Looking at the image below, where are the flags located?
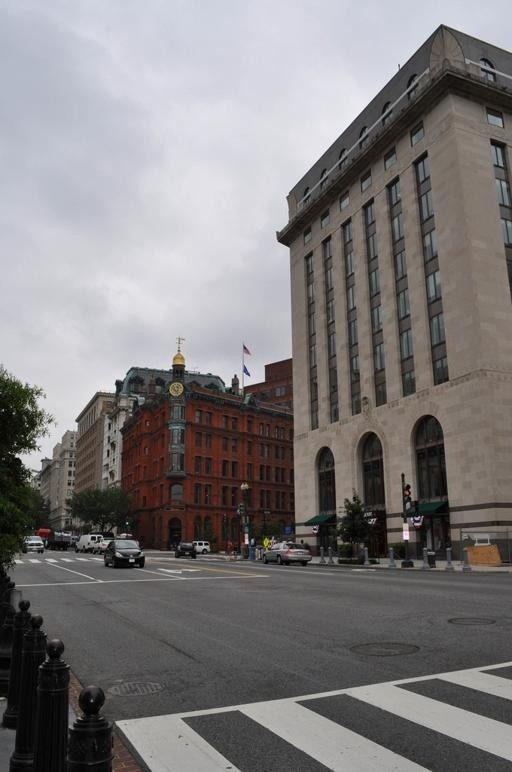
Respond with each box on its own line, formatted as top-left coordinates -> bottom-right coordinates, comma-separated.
243,344 -> 251,377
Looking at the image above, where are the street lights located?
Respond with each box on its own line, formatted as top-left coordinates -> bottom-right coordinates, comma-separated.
239,481 -> 251,558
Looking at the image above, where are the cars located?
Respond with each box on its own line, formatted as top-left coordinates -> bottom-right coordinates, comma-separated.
174,539 -> 210,559
104,540 -> 145,568
263,542 -> 312,566
20,528 -> 113,557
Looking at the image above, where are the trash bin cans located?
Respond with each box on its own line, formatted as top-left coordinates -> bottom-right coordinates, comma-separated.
427,552 -> 436,568
249,547 -> 256,560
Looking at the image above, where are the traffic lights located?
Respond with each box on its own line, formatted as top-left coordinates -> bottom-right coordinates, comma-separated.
403,482 -> 411,503
